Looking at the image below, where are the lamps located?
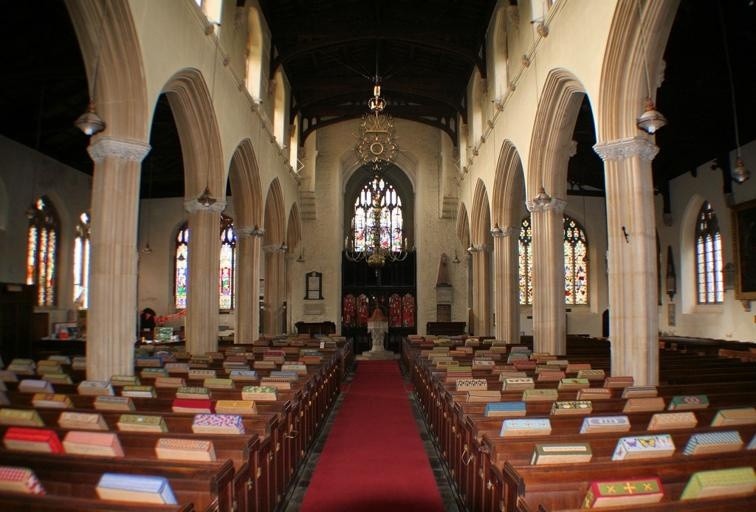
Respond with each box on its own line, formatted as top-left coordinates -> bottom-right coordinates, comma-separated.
525,182 -> 567,213
637,0 -> 666,135
296,224 -> 305,262
488,222 -> 507,239
181,36 -> 227,213
279,241 -> 287,255
466,240 -> 477,254
345,39 -> 416,279
249,101 -> 264,237
74,2 -> 106,136
715,0 -> 750,183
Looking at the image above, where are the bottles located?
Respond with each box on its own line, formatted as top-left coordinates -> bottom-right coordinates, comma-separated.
319,340 -> 325,350
